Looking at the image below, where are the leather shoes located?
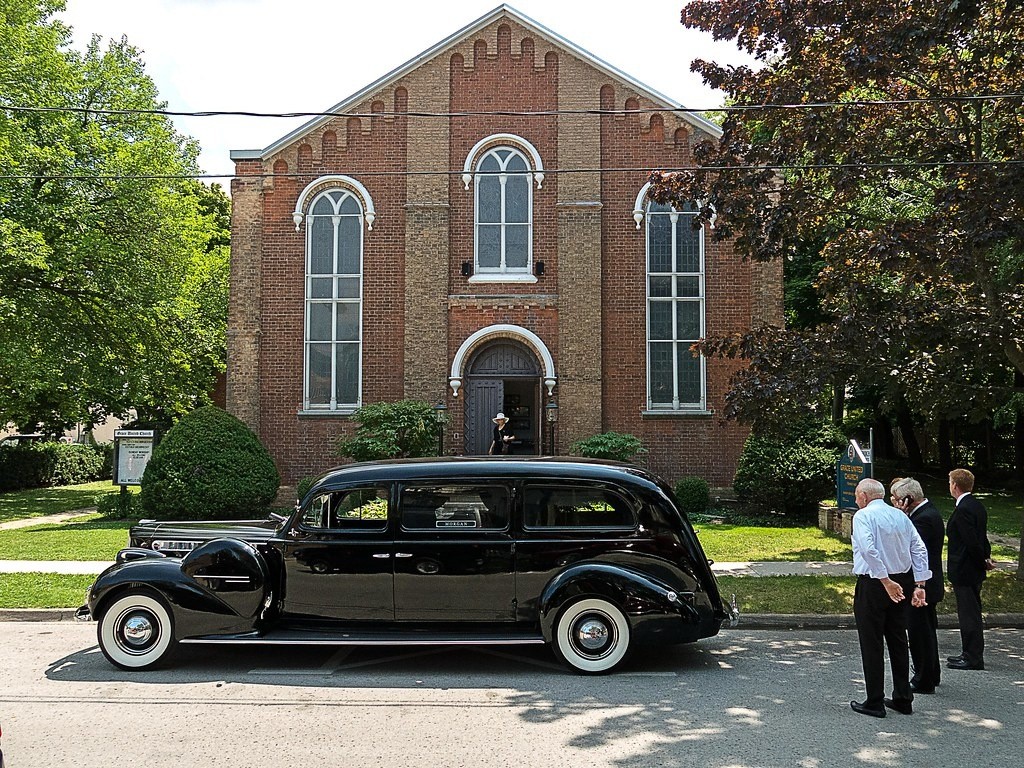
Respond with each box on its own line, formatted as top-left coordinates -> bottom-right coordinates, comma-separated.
948,661 -> 974,670
851,700 -> 886,718
910,664 -> 918,671
909,681 -> 936,694
884,698 -> 903,713
947,655 -> 967,661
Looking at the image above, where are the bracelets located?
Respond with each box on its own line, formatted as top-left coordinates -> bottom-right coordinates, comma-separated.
915,584 -> 925,590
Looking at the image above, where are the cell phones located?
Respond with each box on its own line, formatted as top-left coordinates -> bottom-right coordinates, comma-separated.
902,495 -> 914,508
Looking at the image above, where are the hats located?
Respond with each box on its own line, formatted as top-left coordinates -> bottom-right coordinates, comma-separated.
492,413 -> 509,424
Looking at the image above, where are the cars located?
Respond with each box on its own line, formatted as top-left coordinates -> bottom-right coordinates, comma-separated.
73,453 -> 740,677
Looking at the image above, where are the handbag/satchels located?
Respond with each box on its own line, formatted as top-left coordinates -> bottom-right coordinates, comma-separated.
501,444 -> 509,455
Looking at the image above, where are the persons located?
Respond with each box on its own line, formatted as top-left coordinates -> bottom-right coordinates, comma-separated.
489,412 -> 516,455
946,468 -> 998,670
889,477 -> 946,694
849,478 -> 932,718
